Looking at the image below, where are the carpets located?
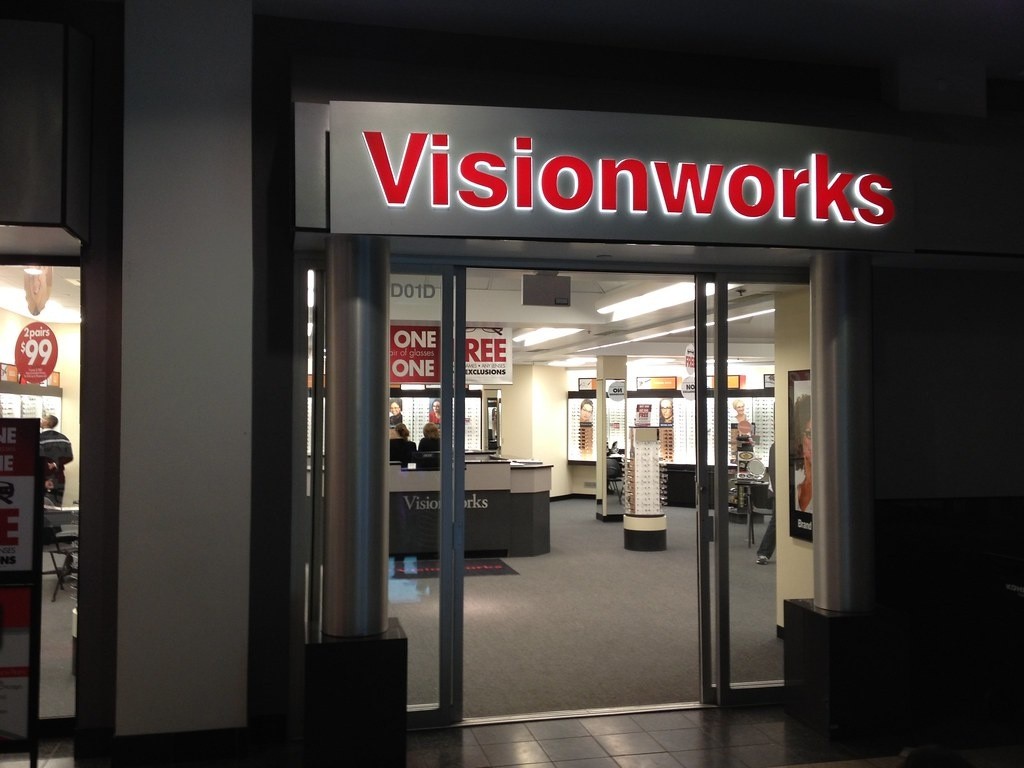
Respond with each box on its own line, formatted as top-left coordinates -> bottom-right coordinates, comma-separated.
387,558 -> 520,580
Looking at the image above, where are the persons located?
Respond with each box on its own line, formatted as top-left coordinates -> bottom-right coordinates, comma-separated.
753,442 -> 775,565
793,392 -> 814,515
731,399 -> 751,424
389,398 -> 404,426
417,422 -> 441,453
429,399 -> 441,439
581,400 -> 593,423
637,404 -> 650,414
389,423 -> 417,453
37,413 -> 74,542
660,398 -> 673,424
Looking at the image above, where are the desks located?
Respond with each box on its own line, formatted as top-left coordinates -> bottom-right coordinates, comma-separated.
44,506 -> 79,526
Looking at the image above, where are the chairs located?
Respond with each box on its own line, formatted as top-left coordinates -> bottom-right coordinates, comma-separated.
43,515 -> 78,602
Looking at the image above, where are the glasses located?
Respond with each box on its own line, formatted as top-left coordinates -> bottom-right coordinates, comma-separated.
677,399 -> 775,462
803,430 -> 811,439
620,407 -> 673,515
569,403 -> 620,455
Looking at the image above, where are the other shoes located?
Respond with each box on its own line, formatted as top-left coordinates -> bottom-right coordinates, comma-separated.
756,556 -> 768,565
